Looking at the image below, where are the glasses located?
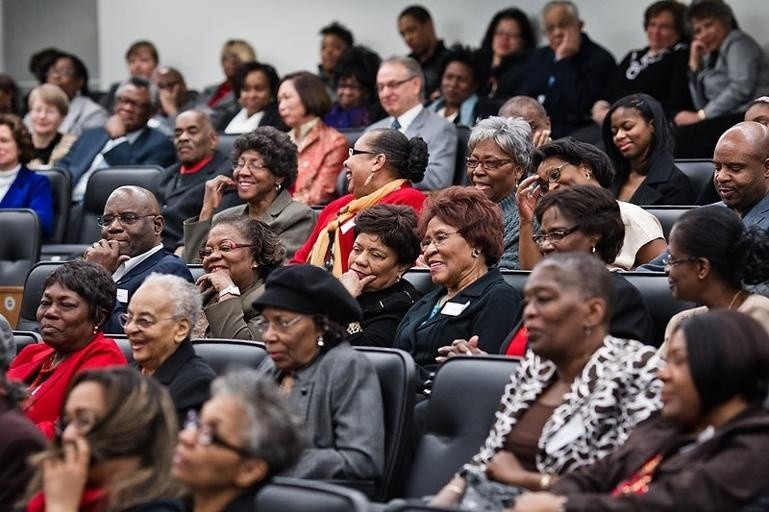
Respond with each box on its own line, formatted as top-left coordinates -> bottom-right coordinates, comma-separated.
374,77 -> 416,90
185,414 -> 242,455
234,162 -> 267,171
250,315 -> 300,332
661,252 -> 697,264
420,230 -> 459,251
200,242 -> 252,257
52,410 -> 97,431
495,30 -> 519,37
96,213 -> 156,228
119,312 -> 169,329
533,224 -> 579,244
535,163 -> 569,196
347,147 -> 374,157
466,158 -> 511,170
542,23 -> 567,29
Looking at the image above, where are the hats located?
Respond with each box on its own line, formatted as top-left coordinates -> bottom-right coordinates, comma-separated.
253,264 -> 363,339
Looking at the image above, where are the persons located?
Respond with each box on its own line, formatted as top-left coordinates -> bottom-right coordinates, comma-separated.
1,2 -> 767,510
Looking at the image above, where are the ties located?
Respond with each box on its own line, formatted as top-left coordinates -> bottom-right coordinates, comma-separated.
391,118 -> 400,130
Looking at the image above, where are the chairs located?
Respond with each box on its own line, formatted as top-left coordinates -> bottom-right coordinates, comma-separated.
353,345 -> 418,502
190,337 -> 267,379
102,334 -> 136,363
406,354 -> 523,500
254,475 -> 364,511
